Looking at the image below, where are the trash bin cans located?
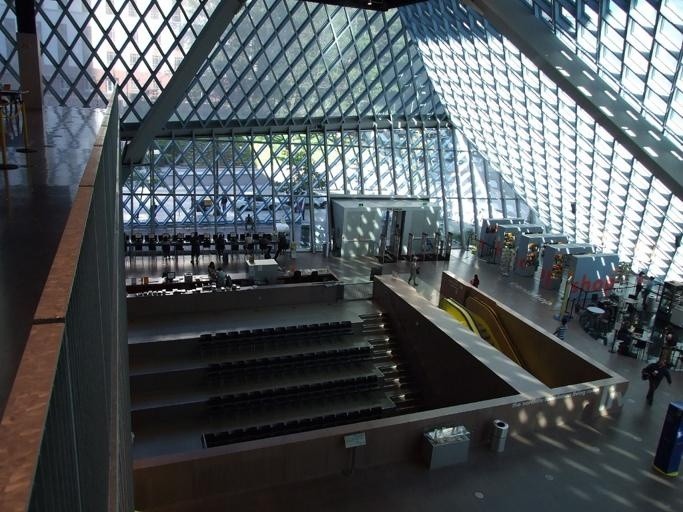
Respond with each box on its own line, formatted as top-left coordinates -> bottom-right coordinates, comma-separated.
323,242 -> 329,257
490,419 -> 509,452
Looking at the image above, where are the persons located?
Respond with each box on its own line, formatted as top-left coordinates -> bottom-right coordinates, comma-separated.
408,256 -> 419,286
617,312 -> 641,347
642,359 -> 672,405
553,318 -> 568,340
472,274 -> 479,287
634,271 -> 654,305
245,214 -> 255,230
243,233 -> 288,260
215,234 -> 225,261
162,237 -> 171,258
208,262 -> 227,288
190,231 -> 200,264
221,197 -> 227,211
659,327 -> 676,365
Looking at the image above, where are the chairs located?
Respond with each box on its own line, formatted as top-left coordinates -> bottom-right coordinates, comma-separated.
199,321 -> 385,450
591,292 -> 637,325
609,327 -> 647,359
293,270 -> 318,282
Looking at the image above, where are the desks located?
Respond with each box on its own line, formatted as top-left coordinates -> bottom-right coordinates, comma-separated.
670,342 -> 683,368
631,335 -> 654,358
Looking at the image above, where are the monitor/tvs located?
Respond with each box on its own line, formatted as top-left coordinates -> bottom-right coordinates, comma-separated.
124,232 -> 272,241
167,272 -> 175,283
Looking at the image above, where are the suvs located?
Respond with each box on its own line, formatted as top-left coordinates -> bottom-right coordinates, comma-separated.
284,190 -> 327,209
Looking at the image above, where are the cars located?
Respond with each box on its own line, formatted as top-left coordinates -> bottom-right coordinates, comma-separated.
235,191 -> 281,211
